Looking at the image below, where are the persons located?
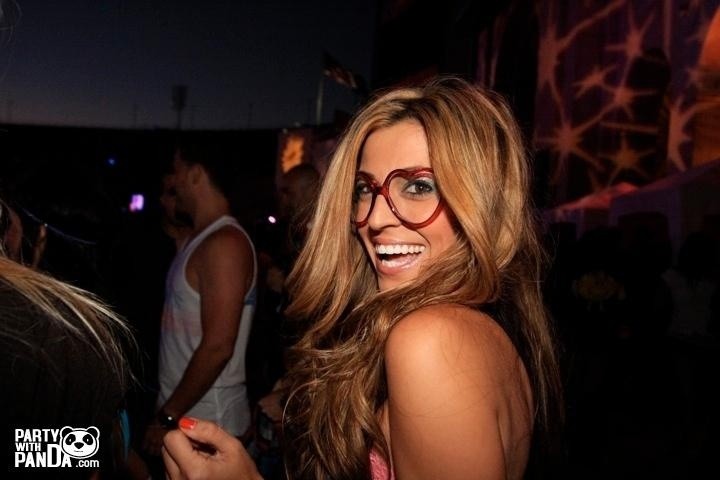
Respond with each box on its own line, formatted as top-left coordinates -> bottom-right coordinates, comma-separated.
159,69 -> 573,480
136,131 -> 340,480
1,240 -> 143,478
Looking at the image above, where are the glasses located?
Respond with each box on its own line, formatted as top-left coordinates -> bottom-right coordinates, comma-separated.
349,168 -> 446,233
